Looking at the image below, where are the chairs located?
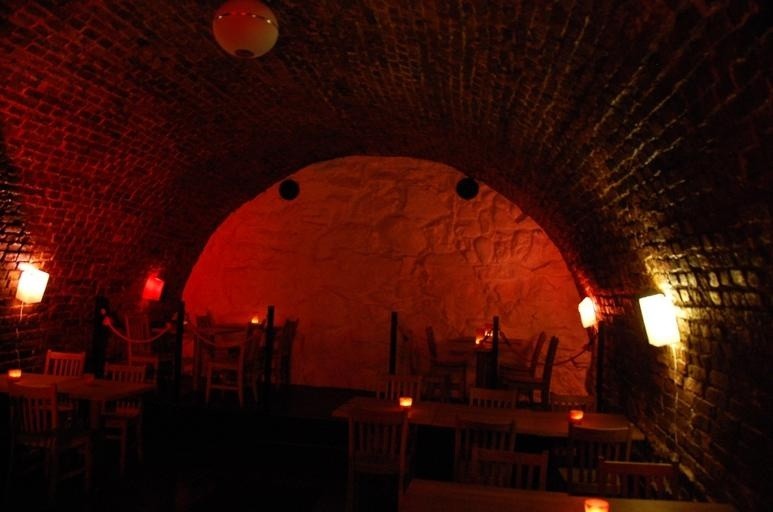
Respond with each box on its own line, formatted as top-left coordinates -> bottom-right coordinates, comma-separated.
446,412 -> 519,486
387,322 -> 565,434
594,454 -> 684,500
168,312 -> 301,406
466,441 -> 549,494
34,346 -> 88,378
342,401 -> 412,500
374,372 -> 425,404
6,379 -> 94,505
92,360 -> 152,468
558,420 -> 637,495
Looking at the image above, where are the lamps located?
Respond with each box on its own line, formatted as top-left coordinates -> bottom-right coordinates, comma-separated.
209,1 -> 281,63
635,292 -> 682,349
11,267 -> 54,307
138,275 -> 166,303
576,295 -> 597,330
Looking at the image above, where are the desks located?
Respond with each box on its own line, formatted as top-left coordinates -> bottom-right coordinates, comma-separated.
396,476 -> 734,510
330,393 -> 649,477
2,369 -> 149,479
